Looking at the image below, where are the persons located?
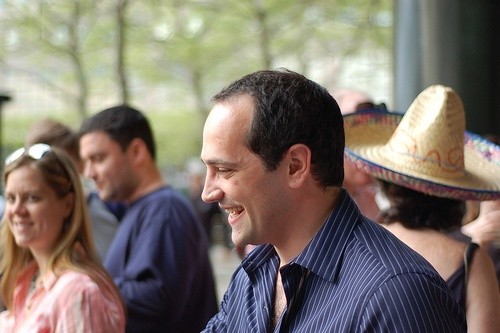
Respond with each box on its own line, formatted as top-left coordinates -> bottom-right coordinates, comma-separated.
0,144 -> 127,333
76,105 -> 221,333
186,153 -> 235,249
199,66 -> 468,333
343,99 -> 500,333
23,117 -> 121,269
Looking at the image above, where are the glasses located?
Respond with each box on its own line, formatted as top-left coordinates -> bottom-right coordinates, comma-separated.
5,143 -> 70,181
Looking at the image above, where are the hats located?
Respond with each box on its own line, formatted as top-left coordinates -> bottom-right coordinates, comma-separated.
26,118 -> 69,146
342,84 -> 500,201
